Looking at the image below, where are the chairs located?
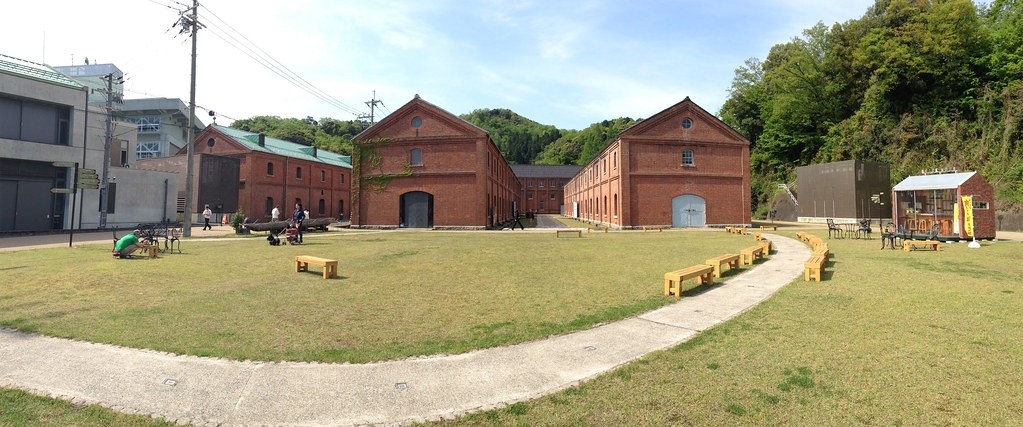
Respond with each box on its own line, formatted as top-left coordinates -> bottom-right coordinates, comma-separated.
136,223 -> 183,254
826,218 -> 845,239
878,221 -> 940,250
858,219 -> 871,238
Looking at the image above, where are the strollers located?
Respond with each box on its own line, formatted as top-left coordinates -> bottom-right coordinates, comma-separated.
282,221 -> 301,245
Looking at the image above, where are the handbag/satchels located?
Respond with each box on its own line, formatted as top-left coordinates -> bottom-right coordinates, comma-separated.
112,250 -> 120,256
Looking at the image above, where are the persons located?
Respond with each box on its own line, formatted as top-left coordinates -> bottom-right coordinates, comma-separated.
202,205 -> 212,230
292,203 -> 305,244
509,209 -> 525,231
525,209 -> 534,227
115,230 -> 147,258
304,209 -> 309,219
272,205 -> 280,222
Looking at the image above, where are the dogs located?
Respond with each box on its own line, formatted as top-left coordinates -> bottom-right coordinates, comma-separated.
267,234 -> 280,246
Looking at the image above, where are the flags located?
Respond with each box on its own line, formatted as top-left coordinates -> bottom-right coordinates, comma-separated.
961,197 -> 973,237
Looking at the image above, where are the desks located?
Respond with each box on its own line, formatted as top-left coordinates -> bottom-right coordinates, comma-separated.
895,234 -> 931,240
835,222 -> 861,238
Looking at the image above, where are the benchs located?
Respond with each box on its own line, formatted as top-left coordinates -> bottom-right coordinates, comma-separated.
588,226 -> 612,233
664,263 -> 714,297
903,239 -> 942,252
133,245 -> 159,257
644,226 -> 663,232
706,253 -> 739,277
796,231 -> 829,267
725,226 -> 772,255
557,229 -> 581,239
803,255 -> 826,281
295,255 -> 338,280
738,245 -> 764,266
